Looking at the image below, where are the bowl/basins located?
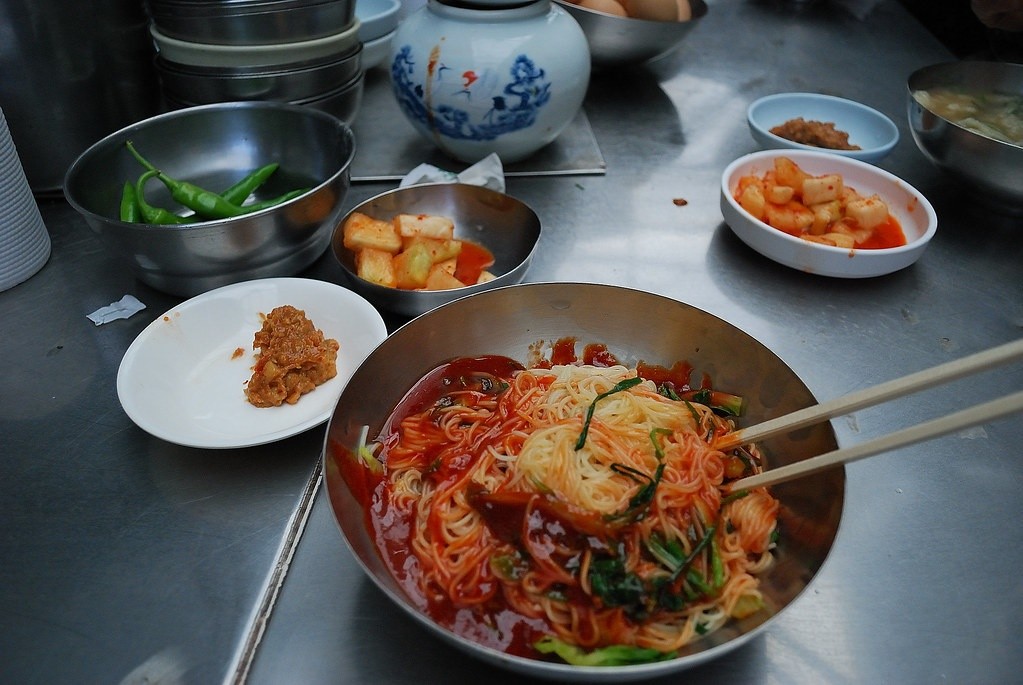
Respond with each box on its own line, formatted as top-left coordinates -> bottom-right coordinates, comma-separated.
719,148 -> 938,279
146,0 -> 402,128
321,281 -> 847,680
551,0 -> 710,67
62,103 -> 358,300
748,93 -> 900,168
908,62 -> 1022,213
329,180 -> 544,321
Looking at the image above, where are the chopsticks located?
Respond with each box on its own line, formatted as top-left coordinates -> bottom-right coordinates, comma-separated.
709,337 -> 1023,494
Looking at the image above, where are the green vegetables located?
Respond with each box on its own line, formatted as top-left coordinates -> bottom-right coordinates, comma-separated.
356,425 -> 382,472
489,376 -> 777,667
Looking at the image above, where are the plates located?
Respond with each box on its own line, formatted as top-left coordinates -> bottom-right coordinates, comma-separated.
114,278 -> 388,450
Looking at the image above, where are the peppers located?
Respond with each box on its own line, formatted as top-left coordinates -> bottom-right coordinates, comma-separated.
119,140 -> 312,224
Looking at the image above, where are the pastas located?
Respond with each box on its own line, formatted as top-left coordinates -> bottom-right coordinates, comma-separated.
384,364 -> 781,651
